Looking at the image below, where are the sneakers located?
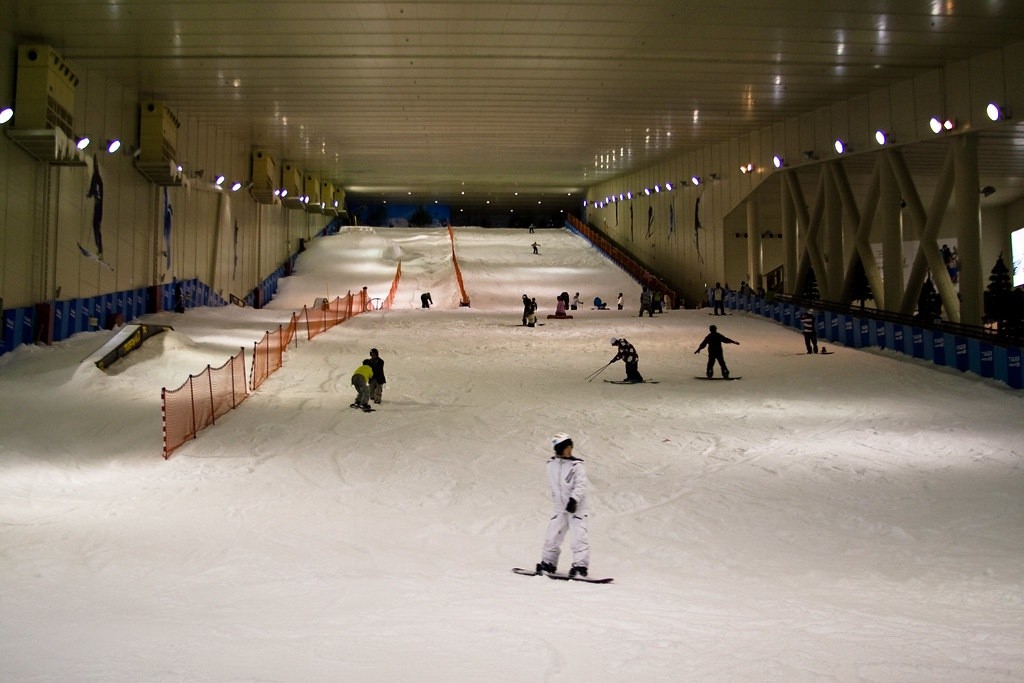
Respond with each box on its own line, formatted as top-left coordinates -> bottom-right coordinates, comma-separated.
536,561 -> 557,574
568,567 -> 587,578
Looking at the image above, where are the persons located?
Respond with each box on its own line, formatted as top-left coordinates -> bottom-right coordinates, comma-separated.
531,242 -> 541,254
556,292 -> 569,316
800,309 -> 818,354
351,359 -> 373,409
536,432 -> 590,576
571,292 -> 583,310
712,282 -> 725,315
694,325 -> 739,378
368,348 -> 386,404
522,294 -> 537,327
725,281 -> 775,303
594,297 -> 607,309
529,224 -> 535,233
617,293 -> 624,310
638,287 -> 662,317
610,338 -> 643,383
421,293 -> 433,308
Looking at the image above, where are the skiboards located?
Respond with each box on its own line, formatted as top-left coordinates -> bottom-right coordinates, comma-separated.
604,379 -> 660,384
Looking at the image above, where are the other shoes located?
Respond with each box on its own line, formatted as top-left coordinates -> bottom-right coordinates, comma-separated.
355,401 -> 361,407
370,396 -> 374,400
360,404 -> 371,409
374,399 -> 381,404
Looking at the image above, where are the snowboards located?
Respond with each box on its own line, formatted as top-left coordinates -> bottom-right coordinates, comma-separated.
350,404 -> 376,412
646,311 -> 669,314
695,377 -> 742,380
708,313 -> 733,316
795,352 -> 835,355
632,316 -> 658,318
591,308 -> 609,310
515,324 -> 545,327
512,568 -> 614,583
532,253 -> 542,256
547,315 -> 573,319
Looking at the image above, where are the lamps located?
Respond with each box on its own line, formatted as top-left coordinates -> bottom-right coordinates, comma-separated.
103,138 -> 121,153
213,173 -> 226,186
980,185 -> 996,197
74,135 -> 91,152
874,128 -> 895,146
772,155 -> 789,168
194,168 -> 204,178
243,180 -> 254,189
985,102 -> 1011,122
834,138 -> 854,153
708,173 -> 720,180
803,150 -> 818,160
583,176 -> 704,208
928,114 -> 956,135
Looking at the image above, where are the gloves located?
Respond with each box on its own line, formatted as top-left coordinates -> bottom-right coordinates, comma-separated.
566,497 -> 577,513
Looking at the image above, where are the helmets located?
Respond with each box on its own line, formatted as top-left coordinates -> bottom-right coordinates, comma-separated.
610,337 -> 617,344
551,432 -> 571,449
370,348 -> 378,356
